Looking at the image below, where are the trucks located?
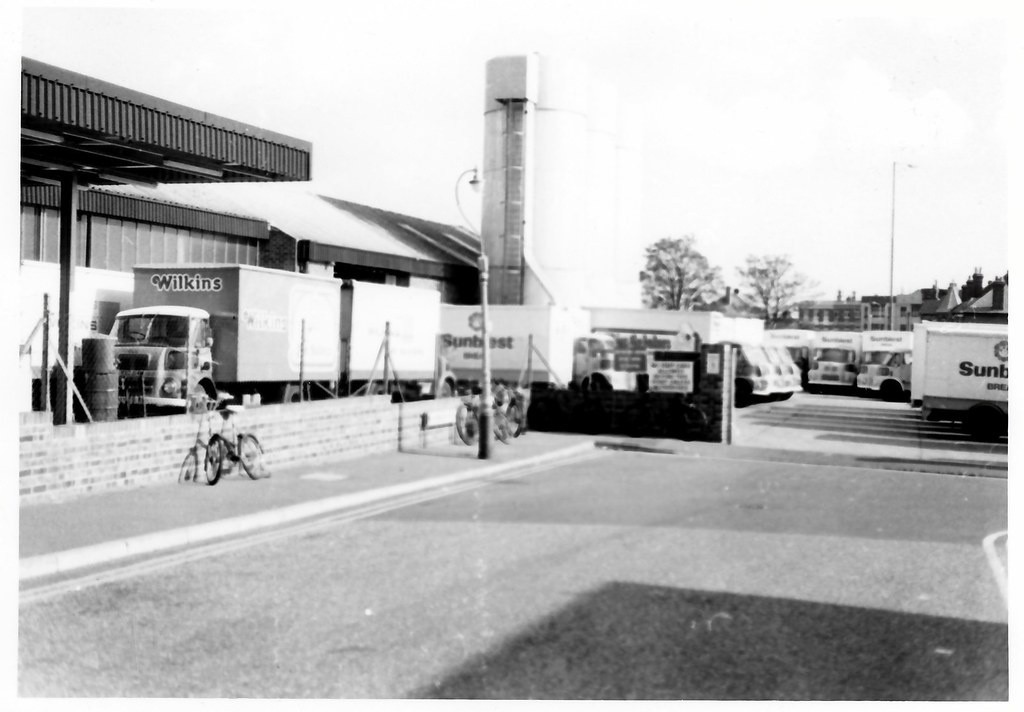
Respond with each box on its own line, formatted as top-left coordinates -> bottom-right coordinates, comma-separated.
438,305 -> 621,391
592,301 -> 1009,445
342,279 -> 457,400
105,261 -> 344,414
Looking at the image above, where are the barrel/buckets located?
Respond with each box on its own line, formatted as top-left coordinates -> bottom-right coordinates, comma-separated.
81,329 -> 122,425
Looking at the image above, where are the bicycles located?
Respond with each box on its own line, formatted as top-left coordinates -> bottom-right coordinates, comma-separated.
204,408 -> 269,486
505,380 -> 530,437
455,380 -> 510,446
